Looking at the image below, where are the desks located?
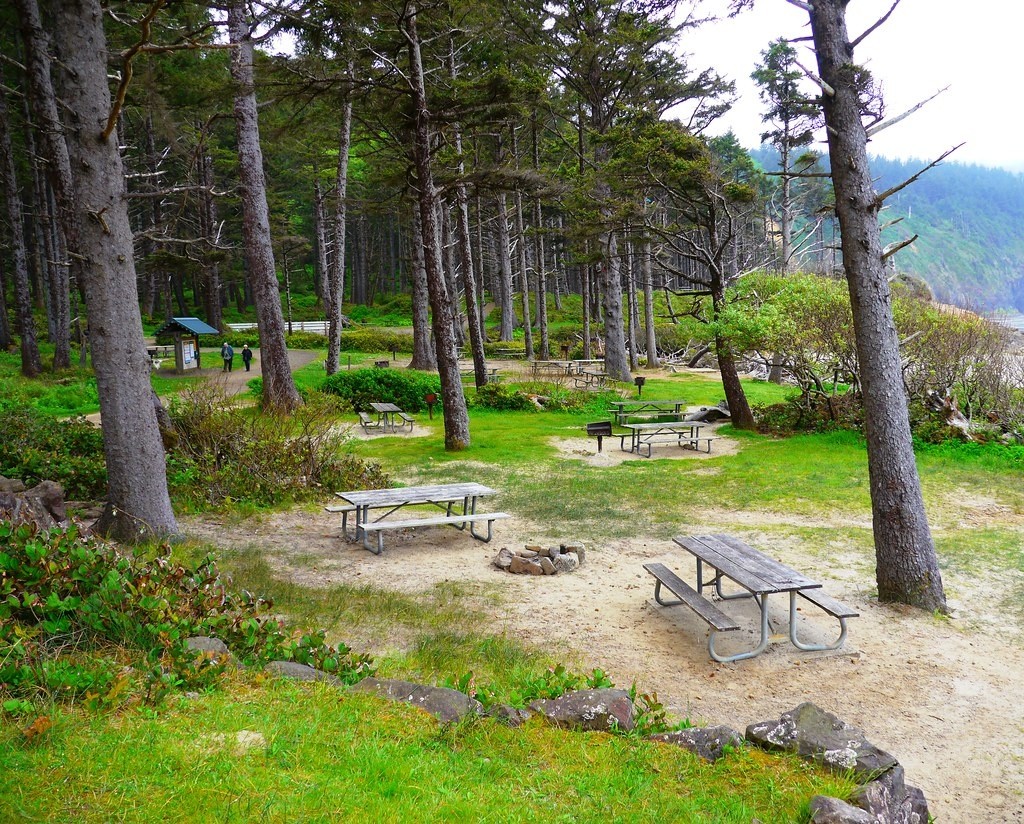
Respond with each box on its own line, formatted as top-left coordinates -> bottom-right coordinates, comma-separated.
370,403 -> 403,433
573,360 -> 605,374
334,483 -> 499,540
581,371 -> 610,389
671,534 -> 823,649
530,360 -> 572,377
621,422 -> 706,451
610,402 -> 686,424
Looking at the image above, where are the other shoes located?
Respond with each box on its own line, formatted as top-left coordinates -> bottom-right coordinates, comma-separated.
229,370 -> 231,372
222,370 -> 227,372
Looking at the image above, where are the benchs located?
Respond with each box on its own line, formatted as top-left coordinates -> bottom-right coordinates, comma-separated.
574,377 -> 618,390
324,495 -> 466,539
606,408 -> 694,426
639,436 -> 721,458
451,346 -> 529,385
359,411 -> 374,435
799,588 -> 860,651
643,561 -> 742,662
613,429 -> 691,452
398,413 -> 415,434
532,363 -> 605,377
357,512 -> 509,554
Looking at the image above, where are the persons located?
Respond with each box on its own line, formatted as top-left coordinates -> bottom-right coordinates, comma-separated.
221,343 -> 233,372
241,345 -> 252,371
